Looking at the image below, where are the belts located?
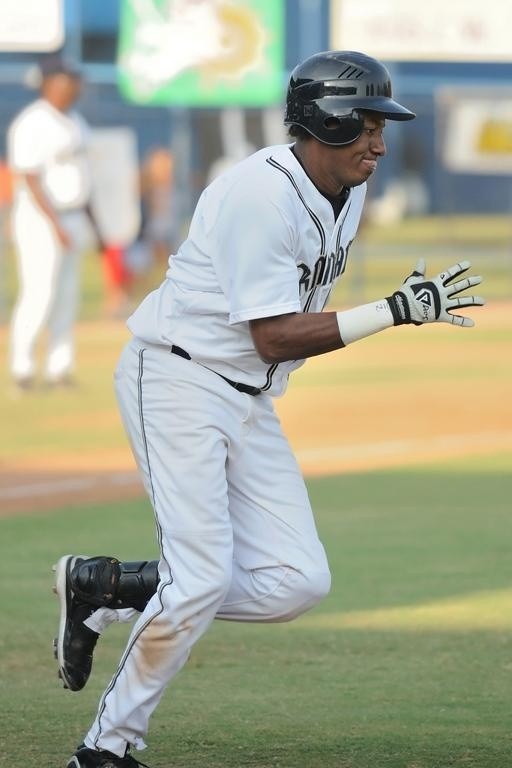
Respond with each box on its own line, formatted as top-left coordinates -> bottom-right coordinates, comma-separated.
171,345 -> 262,396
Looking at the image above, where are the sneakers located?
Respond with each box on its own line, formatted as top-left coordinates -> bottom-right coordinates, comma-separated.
67,743 -> 150,768
52,553 -> 120,692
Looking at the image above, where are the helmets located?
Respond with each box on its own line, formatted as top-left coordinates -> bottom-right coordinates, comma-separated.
284,51 -> 416,146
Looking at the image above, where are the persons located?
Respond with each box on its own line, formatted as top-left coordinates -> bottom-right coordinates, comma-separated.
48,48 -> 491,768
6,60 -> 108,391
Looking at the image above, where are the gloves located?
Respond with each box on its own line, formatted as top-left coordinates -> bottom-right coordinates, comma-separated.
384,258 -> 485,328
